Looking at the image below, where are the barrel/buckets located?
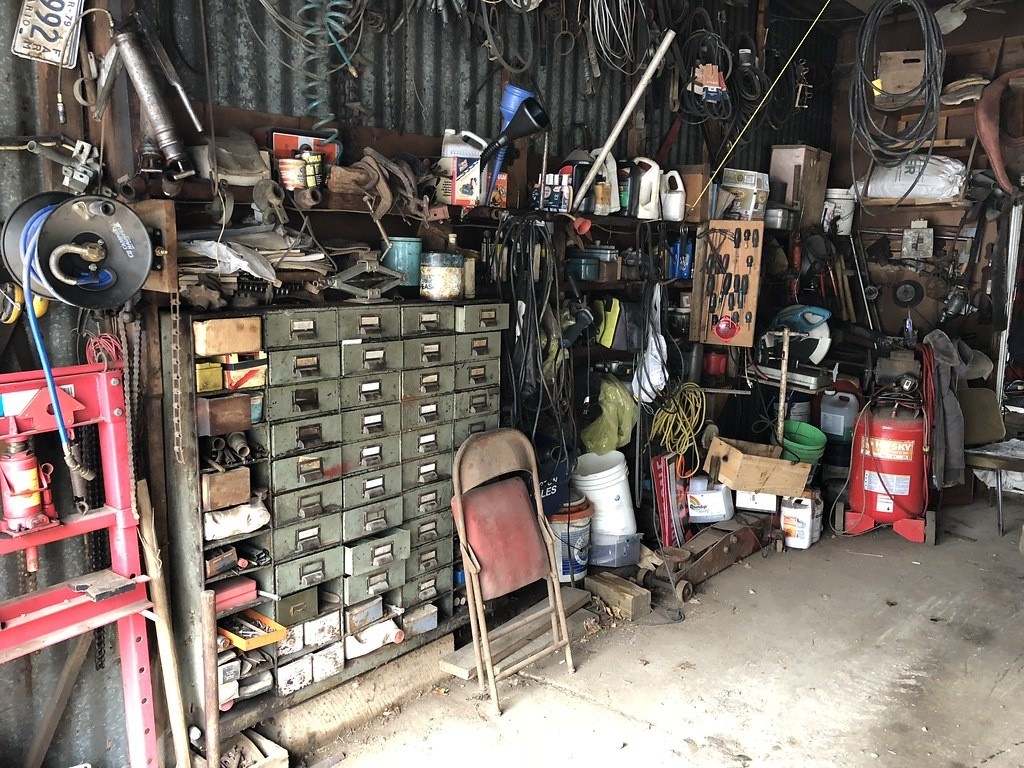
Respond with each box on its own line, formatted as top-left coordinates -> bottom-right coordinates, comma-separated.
819,386 -> 860,447
779,490 -> 824,550
438,128 -> 490,207
558,143 -> 687,223
570,450 -> 637,546
543,489 -> 595,584
769,420 -> 829,491
822,186 -> 857,237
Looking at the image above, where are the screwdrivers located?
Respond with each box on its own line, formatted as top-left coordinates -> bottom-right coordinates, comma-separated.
709,293 -> 716,332
703,253 -> 714,300
704,273 -> 714,342
714,255 -> 732,320
734,228 -> 759,274
718,272 -> 752,331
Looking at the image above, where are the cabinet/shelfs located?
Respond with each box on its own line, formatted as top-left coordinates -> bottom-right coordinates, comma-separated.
860,100 -> 975,204
37,107 -> 799,752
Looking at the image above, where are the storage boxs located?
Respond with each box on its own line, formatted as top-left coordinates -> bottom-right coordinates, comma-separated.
203,729 -> 289,768
205,545 -> 238,579
721,168 -> 770,221
588,533 -> 644,568
278,655 -> 313,698
769,144 -> 832,230
192,316 -> 262,357
277,623 -> 304,656
307,640 -> 344,683
202,466 -> 251,511
196,393 -> 253,437
303,609 -> 341,647
344,594 -> 383,633
665,164 -> 711,223
216,609 -> 288,652
415,156 -> 481,207
564,241 -> 620,282
206,575 -> 257,614
402,604 -> 438,638
876,50 -> 925,100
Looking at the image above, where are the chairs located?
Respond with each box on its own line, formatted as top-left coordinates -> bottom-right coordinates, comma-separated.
935,388 -> 1023,538
450,427 -> 576,717
